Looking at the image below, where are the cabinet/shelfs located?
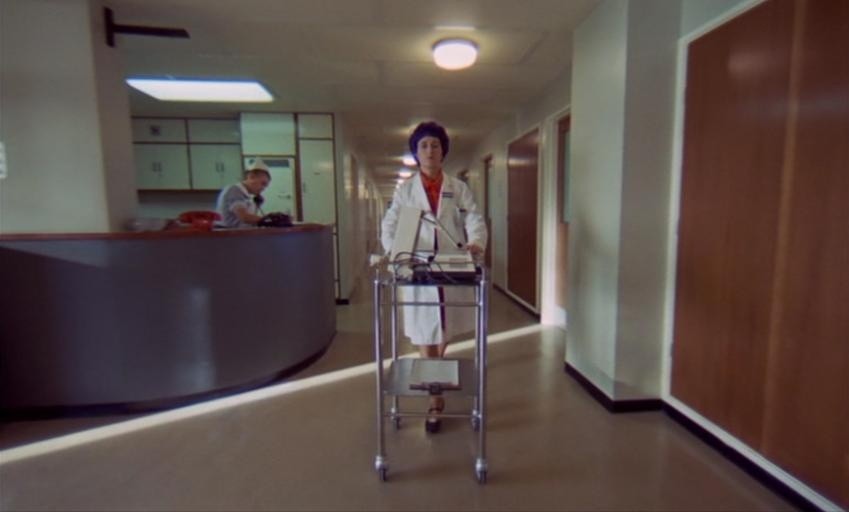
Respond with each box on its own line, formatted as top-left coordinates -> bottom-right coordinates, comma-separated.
187,118 -> 243,191
131,117 -> 191,191
244,157 -> 298,221
297,139 -> 338,234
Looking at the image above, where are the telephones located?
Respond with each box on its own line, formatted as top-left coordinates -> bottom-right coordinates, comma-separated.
253,192 -> 264,203
160,209 -> 222,231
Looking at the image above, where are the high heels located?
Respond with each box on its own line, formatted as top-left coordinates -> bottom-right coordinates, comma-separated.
425,399 -> 445,433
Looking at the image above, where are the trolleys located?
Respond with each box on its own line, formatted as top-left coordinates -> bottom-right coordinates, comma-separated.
371,254 -> 488,488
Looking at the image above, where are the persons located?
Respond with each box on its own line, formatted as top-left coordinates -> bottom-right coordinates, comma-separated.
216,162 -> 272,230
380,120 -> 489,433
